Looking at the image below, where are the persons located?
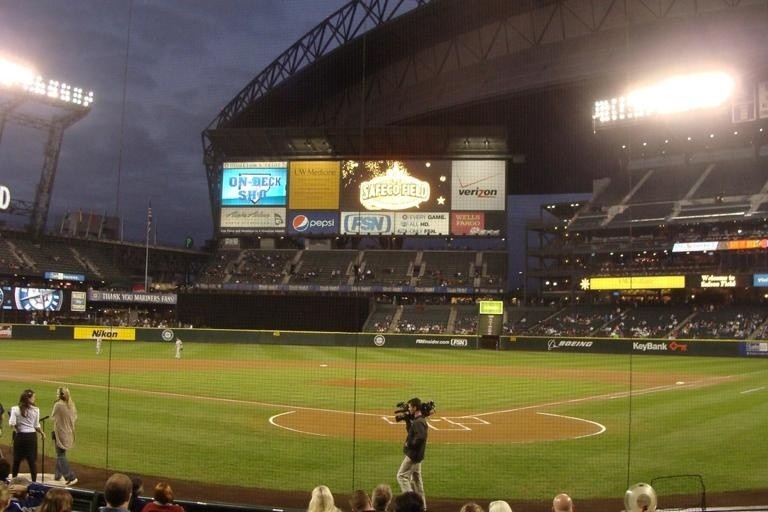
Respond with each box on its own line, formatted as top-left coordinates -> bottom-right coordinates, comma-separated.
27,310 -> 189,328
50,387 -> 78,485
207,264 -> 376,284
521,220 -> 768,340
0,403 -> 4,458
9,389 -> 44,483
377,271 -> 520,336
552,494 -> 575,509
175,337 -> 183,359
307,485 -> 511,512
1,276 -> 129,293
96,334 -> 102,356
0,460 -> 184,512
396,398 -> 428,511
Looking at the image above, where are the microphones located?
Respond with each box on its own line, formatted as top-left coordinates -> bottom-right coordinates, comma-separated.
39,414 -> 50,422
